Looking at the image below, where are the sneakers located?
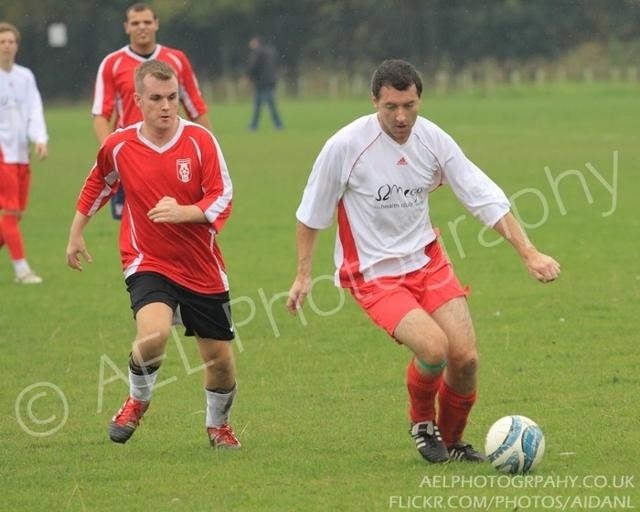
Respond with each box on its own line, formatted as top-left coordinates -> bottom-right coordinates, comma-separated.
16,271 -> 43,285
108,394 -> 151,444
408,418 -> 451,465
207,422 -> 242,452
447,442 -> 488,463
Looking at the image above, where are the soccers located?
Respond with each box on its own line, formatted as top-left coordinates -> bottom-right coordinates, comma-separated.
485,415 -> 546,473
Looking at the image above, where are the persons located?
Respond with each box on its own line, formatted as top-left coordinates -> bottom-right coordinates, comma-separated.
244,34 -> 289,131
0,24 -> 49,286
284,59 -> 563,463
67,2 -> 246,453
